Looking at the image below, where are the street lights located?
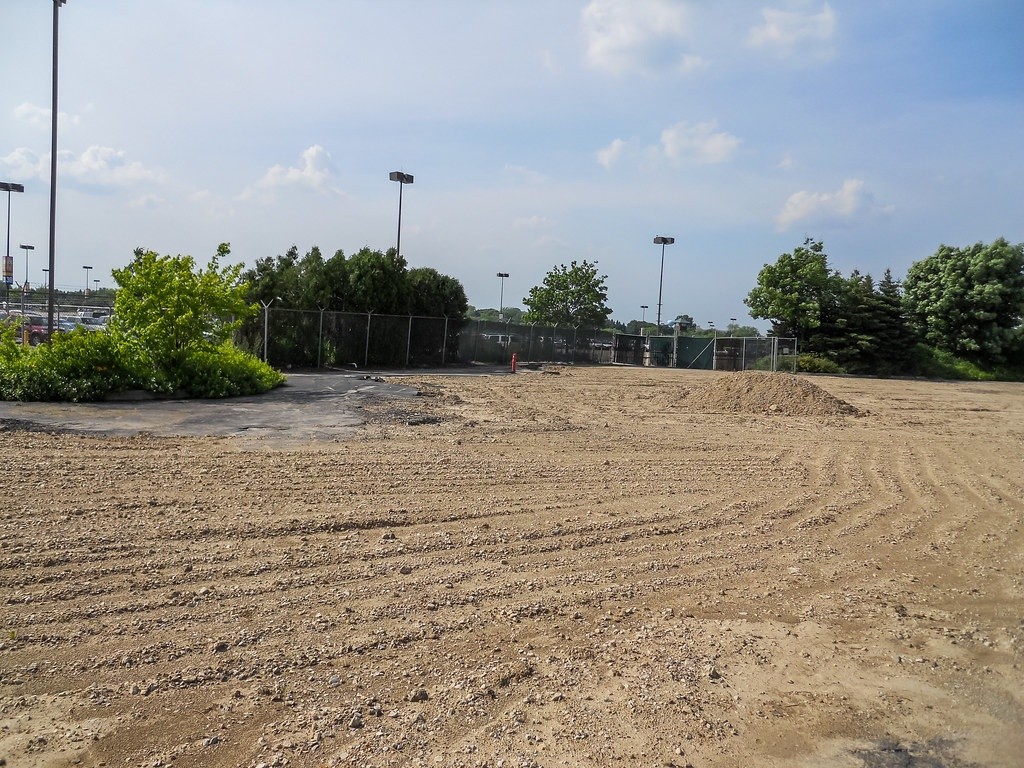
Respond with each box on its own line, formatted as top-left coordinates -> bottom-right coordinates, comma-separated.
94,279 -> 100,291
389,170 -> 414,261
83,265 -> 93,289
640,305 -> 649,328
496,272 -> 510,313
0,182 -> 26,254
19,244 -> 35,300
652,234 -> 675,335
42,268 -> 49,288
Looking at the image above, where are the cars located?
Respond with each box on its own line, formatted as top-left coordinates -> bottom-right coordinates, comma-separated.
0,309 -> 110,347
